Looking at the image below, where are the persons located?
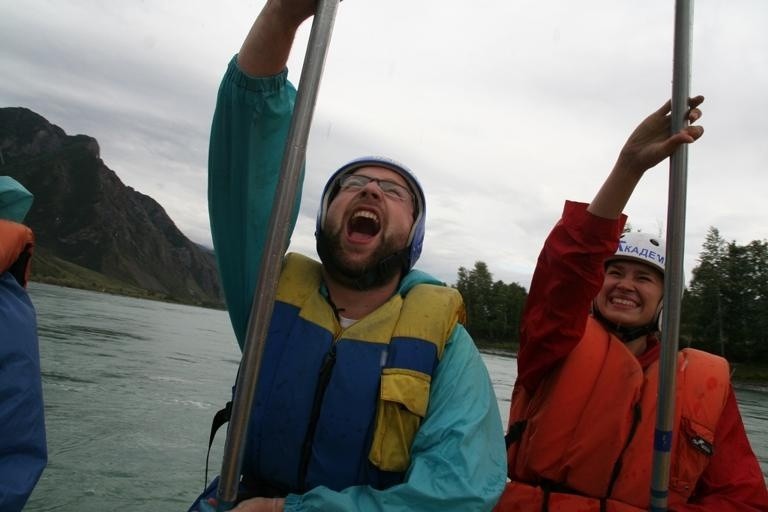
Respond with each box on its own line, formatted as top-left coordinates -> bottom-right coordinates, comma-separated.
498,95 -> 767,511
0,172 -> 48,512
207,0 -> 509,511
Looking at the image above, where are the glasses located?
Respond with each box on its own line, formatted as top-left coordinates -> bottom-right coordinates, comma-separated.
333,173 -> 416,214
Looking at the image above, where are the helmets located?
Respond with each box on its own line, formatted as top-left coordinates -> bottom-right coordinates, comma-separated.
604,231 -> 685,332
314,155 -> 428,275
